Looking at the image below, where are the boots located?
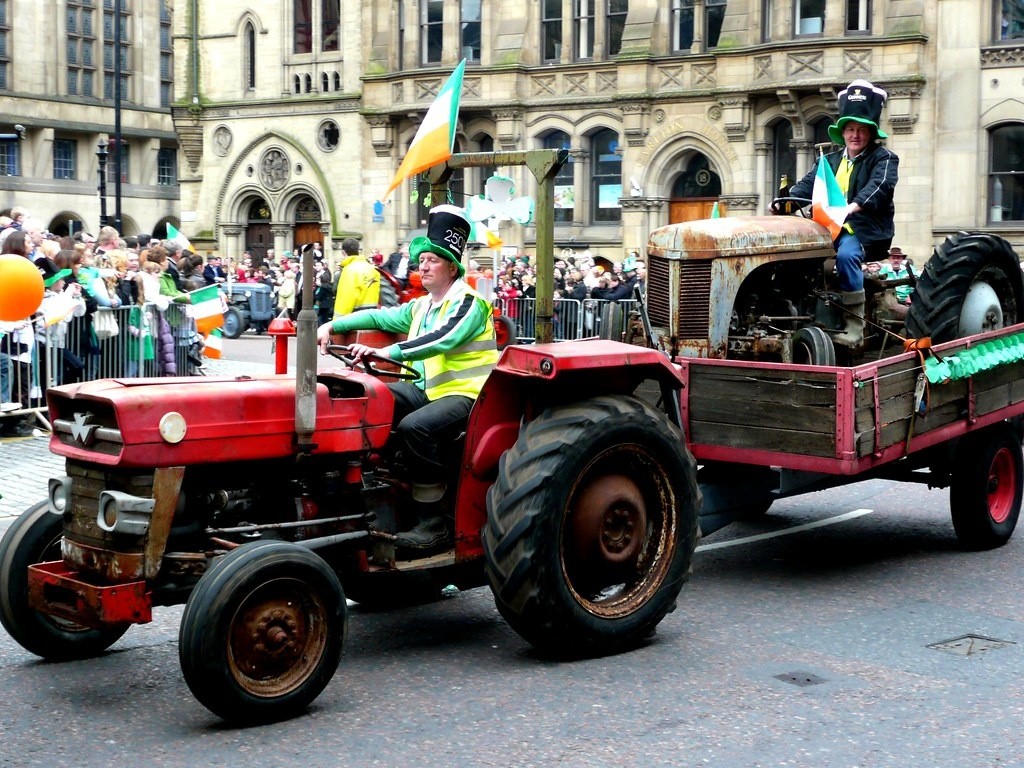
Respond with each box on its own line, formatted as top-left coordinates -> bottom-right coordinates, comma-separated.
832,289 -> 867,349
395,481 -> 451,550
187,343 -> 202,366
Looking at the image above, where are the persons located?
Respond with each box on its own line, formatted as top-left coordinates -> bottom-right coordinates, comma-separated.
316,205 -> 499,552
769,80 -> 900,346
0,208 -> 921,437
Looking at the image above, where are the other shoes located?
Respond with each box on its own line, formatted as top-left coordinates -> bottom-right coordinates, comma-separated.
247,328 -> 256,332
0,401 -> 22,412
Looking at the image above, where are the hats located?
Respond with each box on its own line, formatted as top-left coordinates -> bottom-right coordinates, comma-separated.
507,256 -> 516,263
622,257 -> 638,272
499,275 -> 508,282
866,261 -> 883,267
889,246 -> 908,256
207,255 -> 217,259
244,251 -> 252,255
35,257 -> 73,288
827,79 -> 889,148
408,204 -> 471,277
520,256 -> 529,263
245,259 -> 251,263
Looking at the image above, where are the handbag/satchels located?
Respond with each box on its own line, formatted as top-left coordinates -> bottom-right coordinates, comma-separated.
114,278 -> 139,306
90,309 -> 119,340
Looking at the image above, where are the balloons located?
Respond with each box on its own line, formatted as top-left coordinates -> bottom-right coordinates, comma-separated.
0,254 -> 45,322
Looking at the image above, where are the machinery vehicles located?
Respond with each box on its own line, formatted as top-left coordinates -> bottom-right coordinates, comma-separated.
200,274 -> 274,339
0,146 -> 1022,726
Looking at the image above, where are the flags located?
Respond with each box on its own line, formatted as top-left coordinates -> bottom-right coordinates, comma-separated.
468,219 -> 504,252
811,153 -> 848,240
190,285 -> 224,333
167,223 -> 196,253
383,59 -> 466,202
198,328 -> 223,359
